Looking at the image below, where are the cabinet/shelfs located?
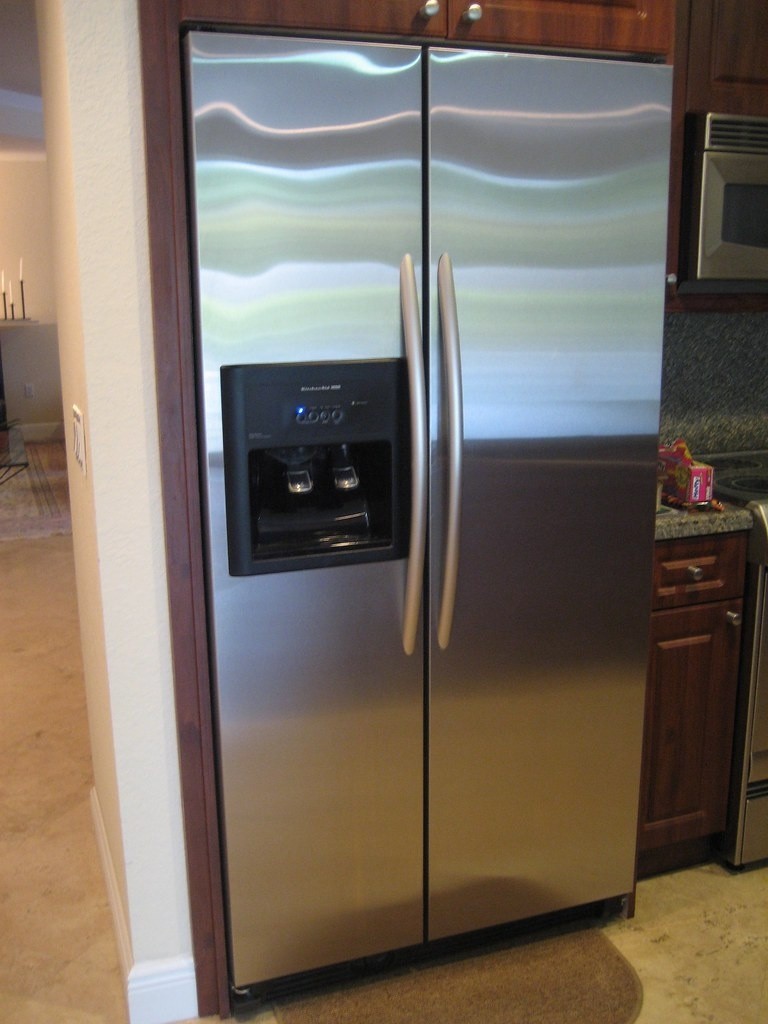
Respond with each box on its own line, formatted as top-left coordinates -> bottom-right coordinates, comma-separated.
634,531 -> 748,880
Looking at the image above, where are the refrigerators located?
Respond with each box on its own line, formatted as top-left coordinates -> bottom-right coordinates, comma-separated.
178,24 -> 676,995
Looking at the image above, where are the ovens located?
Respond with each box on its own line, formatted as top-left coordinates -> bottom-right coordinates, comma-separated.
680,109 -> 767,283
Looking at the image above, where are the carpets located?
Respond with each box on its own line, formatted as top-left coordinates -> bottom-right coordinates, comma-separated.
0,442 -> 72,540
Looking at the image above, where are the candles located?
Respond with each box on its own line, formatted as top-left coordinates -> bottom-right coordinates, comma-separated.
9,280 -> 12,303
2,270 -> 5,292
20,257 -> 23,280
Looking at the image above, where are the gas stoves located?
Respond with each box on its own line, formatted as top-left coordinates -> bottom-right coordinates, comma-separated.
690,445 -> 768,570
